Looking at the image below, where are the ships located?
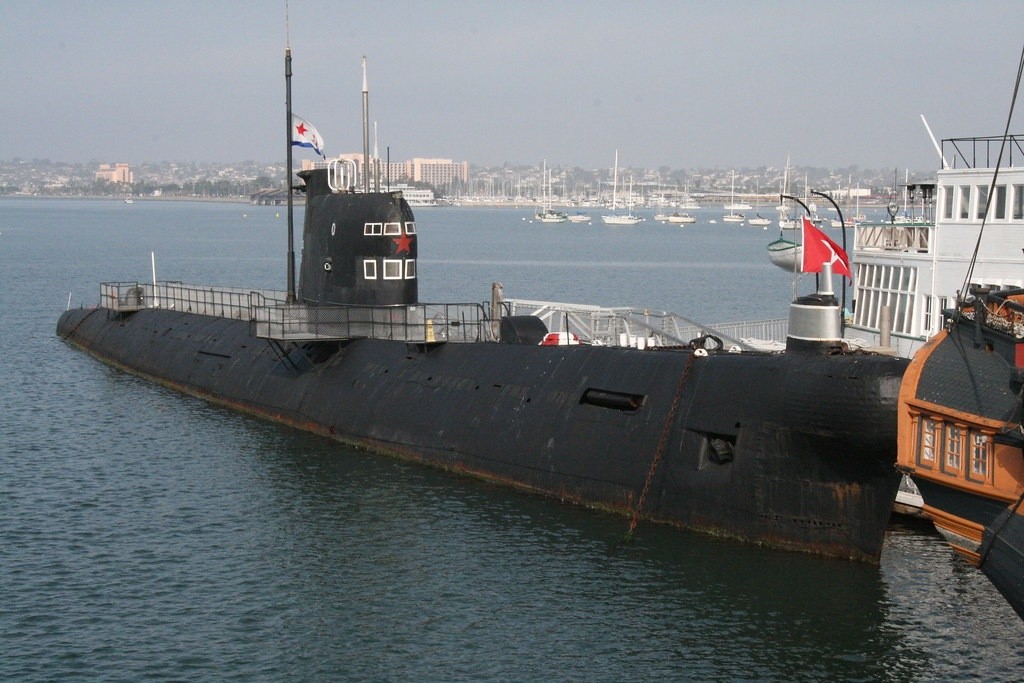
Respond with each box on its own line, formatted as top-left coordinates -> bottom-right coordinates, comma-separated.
54,2 -> 915,565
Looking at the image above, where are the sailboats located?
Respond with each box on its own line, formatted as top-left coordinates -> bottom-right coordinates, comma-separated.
533,149 -> 935,230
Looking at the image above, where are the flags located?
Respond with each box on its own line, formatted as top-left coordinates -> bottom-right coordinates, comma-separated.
290,112 -> 326,160
801,215 -> 852,287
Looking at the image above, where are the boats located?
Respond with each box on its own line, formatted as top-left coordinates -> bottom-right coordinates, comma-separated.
369,183 -> 438,208
896,282 -> 1023,626
726,133 -> 1024,516
